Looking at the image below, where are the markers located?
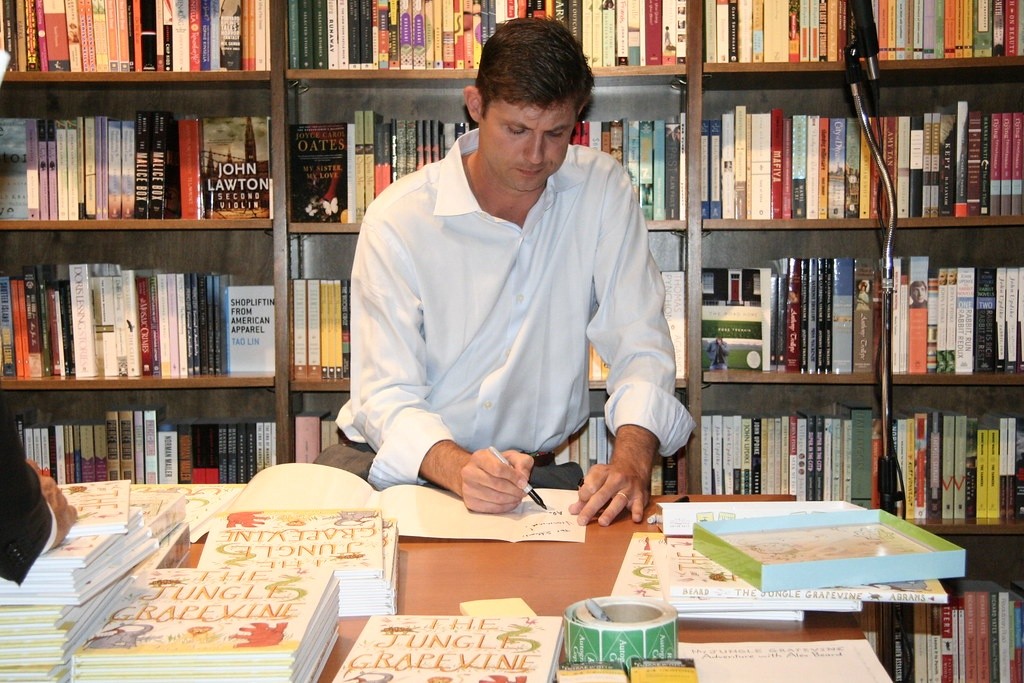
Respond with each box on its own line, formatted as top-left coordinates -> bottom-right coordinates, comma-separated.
489,445 -> 547,511
646,496 -> 689,523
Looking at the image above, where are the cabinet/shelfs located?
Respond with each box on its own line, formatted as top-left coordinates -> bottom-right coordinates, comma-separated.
0,0 -> 283,494
278,0 -> 694,494
694,0 -> 1024,683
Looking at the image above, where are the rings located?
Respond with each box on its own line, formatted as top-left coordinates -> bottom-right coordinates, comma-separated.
616,492 -> 630,503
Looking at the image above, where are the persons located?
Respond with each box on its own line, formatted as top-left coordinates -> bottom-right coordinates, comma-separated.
336,19 -> 697,525
0,397 -> 77,588
909,281 -> 927,309
705,337 -> 729,371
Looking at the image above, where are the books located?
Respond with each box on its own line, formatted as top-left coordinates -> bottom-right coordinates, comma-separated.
0,103 -> 1023,225
857,577 -> 1023,683
17,408 -> 1024,525
0,252 -> 1024,379
0,482 -> 969,683
3,2 -> 1022,75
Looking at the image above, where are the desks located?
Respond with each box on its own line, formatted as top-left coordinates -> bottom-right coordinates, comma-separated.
0,494 -> 896,683
